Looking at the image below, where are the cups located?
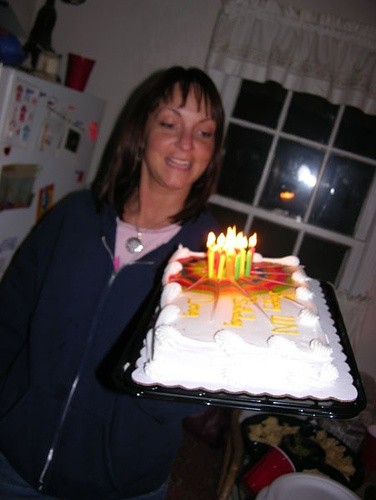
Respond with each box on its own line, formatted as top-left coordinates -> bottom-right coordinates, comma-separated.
236,446 -> 296,497
362,424 -> 376,472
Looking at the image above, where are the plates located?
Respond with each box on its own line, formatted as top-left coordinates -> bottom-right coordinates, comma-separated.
256,471 -> 355,495
241,413 -> 366,492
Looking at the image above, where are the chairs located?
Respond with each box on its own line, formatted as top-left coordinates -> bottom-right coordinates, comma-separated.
216,405 -> 364,500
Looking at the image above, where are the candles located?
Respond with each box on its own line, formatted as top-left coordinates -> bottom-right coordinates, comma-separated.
207,227 -> 256,280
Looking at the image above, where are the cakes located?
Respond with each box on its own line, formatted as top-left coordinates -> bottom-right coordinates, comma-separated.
132,242 -> 359,403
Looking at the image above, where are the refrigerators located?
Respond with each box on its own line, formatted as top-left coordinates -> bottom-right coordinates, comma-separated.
1,66 -> 108,284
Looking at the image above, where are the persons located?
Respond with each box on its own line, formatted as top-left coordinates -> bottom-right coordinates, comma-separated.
0,65 -> 227,500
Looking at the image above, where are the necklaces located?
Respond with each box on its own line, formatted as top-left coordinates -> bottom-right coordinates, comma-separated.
124,183 -> 193,255
